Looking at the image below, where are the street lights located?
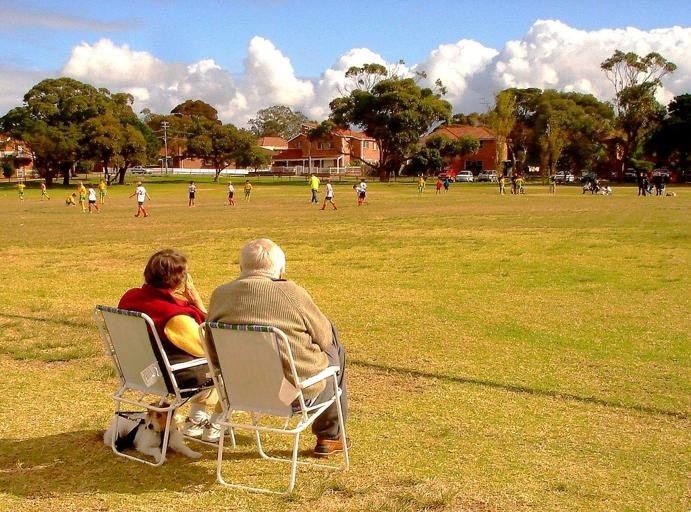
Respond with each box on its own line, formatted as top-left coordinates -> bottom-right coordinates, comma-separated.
158,159 -> 164,173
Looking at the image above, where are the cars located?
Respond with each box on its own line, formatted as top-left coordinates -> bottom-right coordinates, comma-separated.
551,166 -> 679,183
132,165 -> 148,174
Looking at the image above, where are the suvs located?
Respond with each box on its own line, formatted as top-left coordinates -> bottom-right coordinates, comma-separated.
479,170 -> 499,182
456,170 -> 475,182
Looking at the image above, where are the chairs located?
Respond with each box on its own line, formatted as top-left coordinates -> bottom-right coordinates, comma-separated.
93,302 -> 238,467
197,318 -> 352,496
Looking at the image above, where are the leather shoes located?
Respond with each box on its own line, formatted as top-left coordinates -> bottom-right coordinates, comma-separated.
315,435 -> 354,456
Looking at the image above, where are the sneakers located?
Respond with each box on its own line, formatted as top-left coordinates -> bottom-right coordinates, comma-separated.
320,208 -> 326,211
228,203 -> 236,206
144,212 -> 149,218
134,214 -> 141,218
201,420 -> 235,442
332,208 -> 337,210
181,415 -> 211,437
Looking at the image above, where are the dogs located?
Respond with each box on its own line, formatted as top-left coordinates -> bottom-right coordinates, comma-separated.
101,401 -> 202,463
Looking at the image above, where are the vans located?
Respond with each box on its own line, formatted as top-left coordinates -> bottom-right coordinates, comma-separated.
439,169 -> 458,183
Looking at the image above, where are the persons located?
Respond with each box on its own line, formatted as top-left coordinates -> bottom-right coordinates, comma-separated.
581,175 -> 611,195
495,171 -> 525,198
637,168 -> 667,198
205,237 -> 352,457
76,181 -> 87,214
435,178 -> 443,197
187,180 -> 198,208
415,176 -> 426,199
64,192 -> 77,208
548,173 -> 556,192
128,181 -> 152,218
97,178 -> 107,204
226,180 -> 236,207
444,179 -> 451,195
120,250 -> 235,442
39,181 -> 51,202
308,173 -> 321,205
241,180 -> 254,203
359,180 -> 369,206
86,183 -> 101,214
16,181 -> 27,203
353,185 -> 366,208
319,180 -> 338,211
